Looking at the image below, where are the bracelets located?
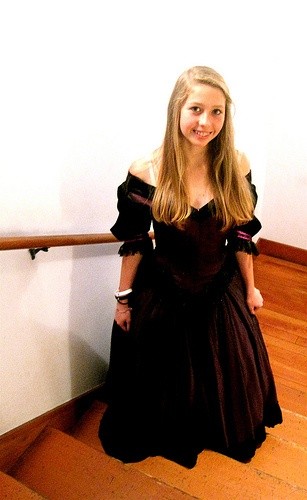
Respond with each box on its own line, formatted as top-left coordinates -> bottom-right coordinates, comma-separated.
115,295 -> 129,300
117,299 -> 129,305
114,288 -> 132,296
115,307 -> 132,313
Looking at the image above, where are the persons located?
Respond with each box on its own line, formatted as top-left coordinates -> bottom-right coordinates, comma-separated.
110,67 -> 266,445
